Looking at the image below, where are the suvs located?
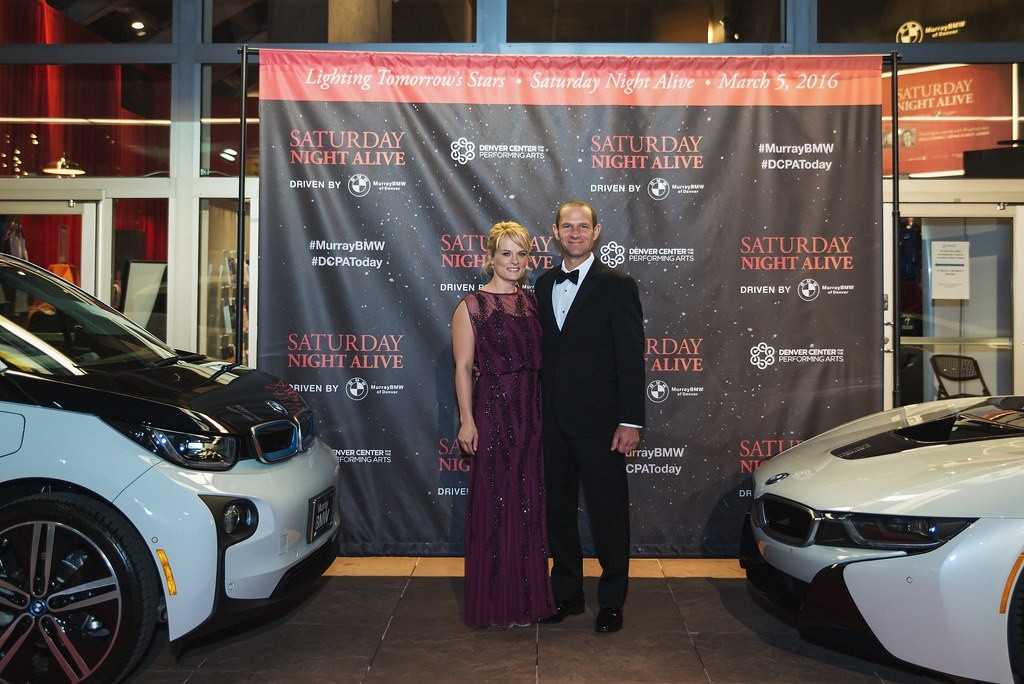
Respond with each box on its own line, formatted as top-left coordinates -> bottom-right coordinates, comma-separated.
0,252 -> 342,684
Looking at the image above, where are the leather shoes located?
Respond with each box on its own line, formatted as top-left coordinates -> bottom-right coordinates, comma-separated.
540,605 -> 585,624
596,605 -> 623,633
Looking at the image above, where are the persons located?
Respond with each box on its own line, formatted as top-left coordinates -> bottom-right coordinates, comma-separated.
533,201 -> 646,634
452,221 -> 558,628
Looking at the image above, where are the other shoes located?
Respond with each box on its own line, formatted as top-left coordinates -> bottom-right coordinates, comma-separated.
490,622 -> 531,628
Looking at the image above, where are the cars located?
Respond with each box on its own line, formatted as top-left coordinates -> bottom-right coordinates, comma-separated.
738,393 -> 1024,684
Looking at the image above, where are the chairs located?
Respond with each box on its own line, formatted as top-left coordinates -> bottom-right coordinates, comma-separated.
929,354 -> 991,400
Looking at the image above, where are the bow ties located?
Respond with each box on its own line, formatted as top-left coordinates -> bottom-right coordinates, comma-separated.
556,269 -> 579,285
